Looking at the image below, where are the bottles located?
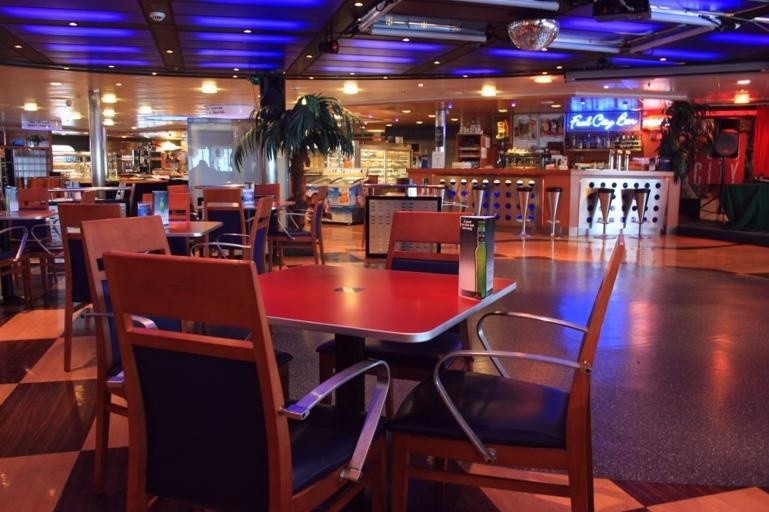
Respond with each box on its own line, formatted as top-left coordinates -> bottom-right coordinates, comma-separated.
6,187 -> 17,216
152,191 -> 170,226
473,221 -> 486,299
567,133 -> 639,149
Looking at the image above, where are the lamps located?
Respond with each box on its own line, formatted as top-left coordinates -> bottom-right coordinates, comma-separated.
507,18 -> 560,52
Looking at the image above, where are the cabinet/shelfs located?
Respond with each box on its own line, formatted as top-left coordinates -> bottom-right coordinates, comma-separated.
459,146 -> 480,159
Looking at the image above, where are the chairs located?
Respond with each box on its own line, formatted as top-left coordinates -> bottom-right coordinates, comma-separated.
314,211 -> 477,403
386,236 -> 626,512
101,249 -> 391,512
57,203 -> 123,372
1,177 -> 625,235
188,196 -> 272,273
274,184 -> 327,265
1,226 -> 30,309
79,214 -> 294,485
30,223 -> 65,294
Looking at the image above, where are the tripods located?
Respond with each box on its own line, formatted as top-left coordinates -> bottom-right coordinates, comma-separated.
699,157 -> 729,223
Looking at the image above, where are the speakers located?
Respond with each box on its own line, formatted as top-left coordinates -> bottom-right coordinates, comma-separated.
712,118 -> 738,158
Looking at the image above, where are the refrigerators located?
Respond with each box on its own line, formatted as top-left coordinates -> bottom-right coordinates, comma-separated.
305,176 -> 367,225
341,140 -> 412,184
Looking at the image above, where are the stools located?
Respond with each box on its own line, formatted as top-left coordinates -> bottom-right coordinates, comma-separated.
620,187 -> 648,240
